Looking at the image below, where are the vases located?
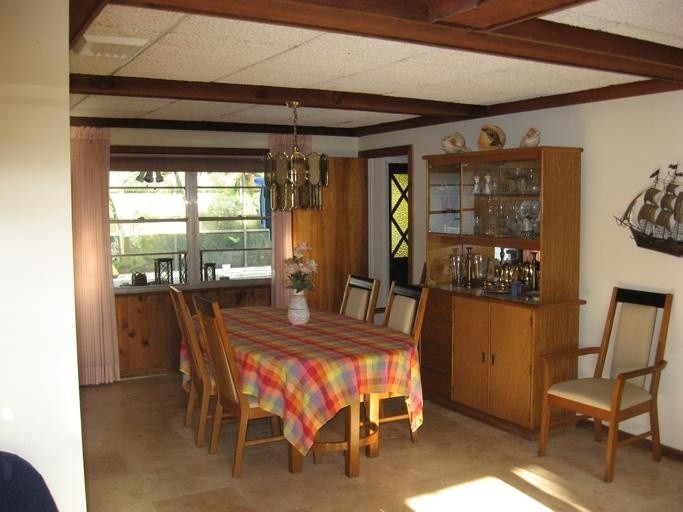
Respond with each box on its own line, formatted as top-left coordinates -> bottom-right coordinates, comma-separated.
284,289 -> 312,326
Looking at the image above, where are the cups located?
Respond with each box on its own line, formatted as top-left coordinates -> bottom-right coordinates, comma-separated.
511,281 -> 524,296
450,254 -> 494,285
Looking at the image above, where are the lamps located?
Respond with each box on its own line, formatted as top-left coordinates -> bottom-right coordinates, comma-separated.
260,100 -> 330,215
134,167 -> 165,185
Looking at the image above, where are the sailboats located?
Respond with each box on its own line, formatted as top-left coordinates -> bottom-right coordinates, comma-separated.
611,162 -> 683,257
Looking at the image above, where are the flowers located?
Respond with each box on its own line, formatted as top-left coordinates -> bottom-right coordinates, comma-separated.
278,241 -> 321,293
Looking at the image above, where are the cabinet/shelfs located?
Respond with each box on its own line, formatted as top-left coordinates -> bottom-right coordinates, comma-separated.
419,144 -> 583,442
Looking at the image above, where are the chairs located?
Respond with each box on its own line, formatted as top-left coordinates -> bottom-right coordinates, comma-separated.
539,284 -> 674,481
190,295 -> 303,479
365,282 -> 431,459
339,273 -> 381,324
167,285 -> 283,456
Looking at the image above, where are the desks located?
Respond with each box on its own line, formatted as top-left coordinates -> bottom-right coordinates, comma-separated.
169,303 -> 424,479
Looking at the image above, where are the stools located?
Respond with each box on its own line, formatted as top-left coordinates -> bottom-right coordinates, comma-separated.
153,257 -> 218,282
